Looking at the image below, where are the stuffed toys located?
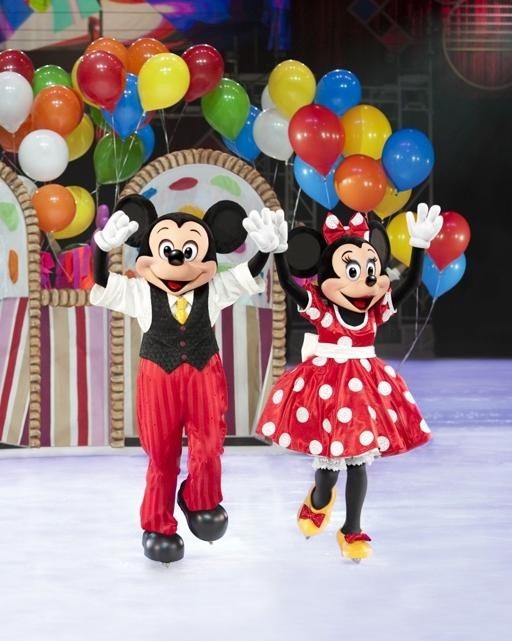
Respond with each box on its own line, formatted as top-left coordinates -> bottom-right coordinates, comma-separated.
255,201 -> 445,561
87,194 -> 281,563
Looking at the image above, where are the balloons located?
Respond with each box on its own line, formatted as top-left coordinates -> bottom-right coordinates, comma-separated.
254,59 -> 435,218
30,184 -> 96,238
387,211 -> 469,299
181,44 -> 224,101
201,77 -> 262,161
0,36 -> 189,186
